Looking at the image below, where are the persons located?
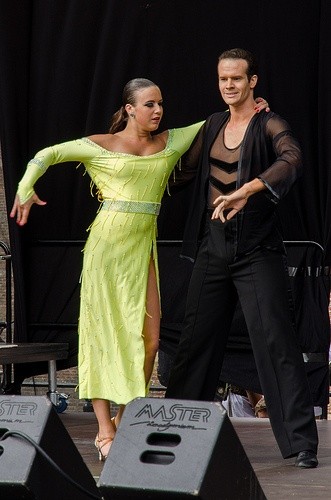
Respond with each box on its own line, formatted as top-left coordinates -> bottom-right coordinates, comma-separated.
254,397 -> 270,419
94,48 -> 320,468
8,79 -> 272,464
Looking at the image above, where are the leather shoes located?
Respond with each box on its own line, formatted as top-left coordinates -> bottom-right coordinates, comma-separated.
296,450 -> 319,468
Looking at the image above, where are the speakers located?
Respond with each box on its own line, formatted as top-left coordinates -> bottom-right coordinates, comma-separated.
97,397 -> 270,500
0,394 -> 100,500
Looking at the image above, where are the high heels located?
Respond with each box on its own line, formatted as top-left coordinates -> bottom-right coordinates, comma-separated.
94,432 -> 115,462
